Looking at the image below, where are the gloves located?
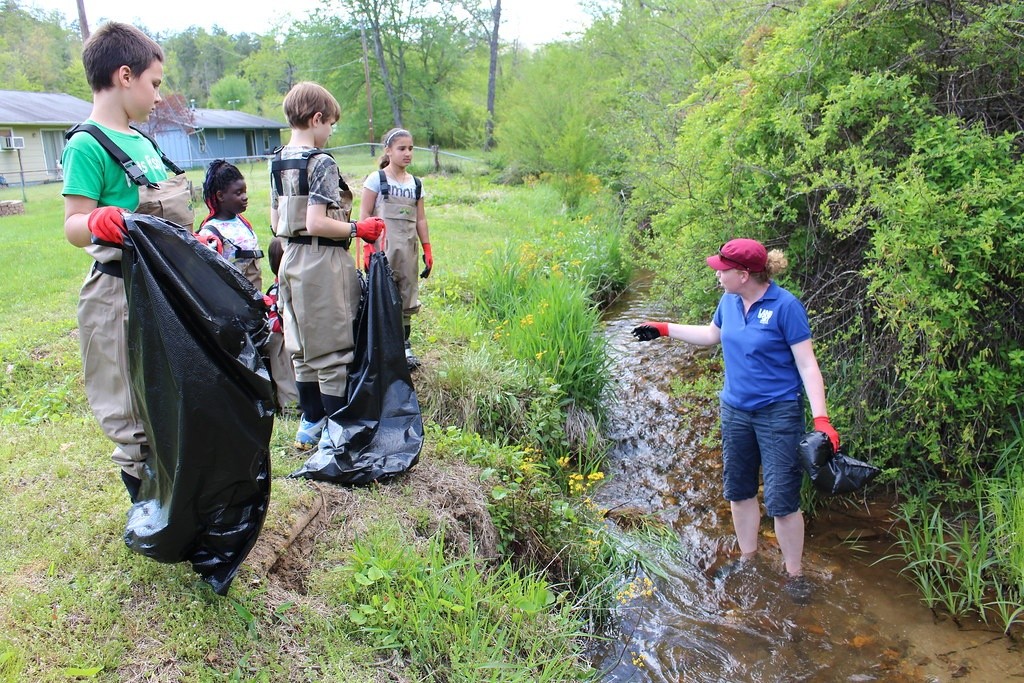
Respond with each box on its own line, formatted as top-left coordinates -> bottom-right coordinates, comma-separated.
353,216 -> 386,243
630,321 -> 669,342
87,205 -> 133,244
813,417 -> 843,453
422,243 -> 435,278
362,242 -> 378,270
195,233 -> 222,255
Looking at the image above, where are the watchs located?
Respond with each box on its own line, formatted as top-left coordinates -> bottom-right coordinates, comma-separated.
350,222 -> 357,238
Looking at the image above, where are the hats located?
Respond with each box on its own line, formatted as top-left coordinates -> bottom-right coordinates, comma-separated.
705,237 -> 768,273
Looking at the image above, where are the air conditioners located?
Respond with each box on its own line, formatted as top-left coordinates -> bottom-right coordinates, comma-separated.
2,136 -> 24,149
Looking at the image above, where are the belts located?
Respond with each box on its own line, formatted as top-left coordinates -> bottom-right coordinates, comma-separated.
285,236 -> 352,248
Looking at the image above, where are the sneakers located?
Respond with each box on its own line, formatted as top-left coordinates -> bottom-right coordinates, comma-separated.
293,412 -> 325,451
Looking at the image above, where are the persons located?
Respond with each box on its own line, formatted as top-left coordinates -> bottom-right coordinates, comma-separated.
630,238 -> 839,580
262,239 -> 300,413
355,125 -> 434,367
266,82 -> 448,527
62,19 -> 222,498
197,157 -> 265,291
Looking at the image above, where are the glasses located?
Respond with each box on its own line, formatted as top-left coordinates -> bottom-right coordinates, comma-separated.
717,244 -> 751,271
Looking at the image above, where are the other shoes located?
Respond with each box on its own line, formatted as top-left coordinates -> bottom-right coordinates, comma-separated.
784,571 -> 813,603
716,552 -> 764,579
404,340 -> 425,370
